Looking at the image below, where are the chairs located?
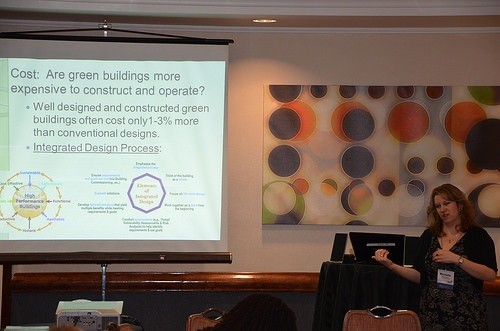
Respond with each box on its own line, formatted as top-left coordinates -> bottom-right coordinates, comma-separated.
186,308 -> 228,331
342,306 -> 422,331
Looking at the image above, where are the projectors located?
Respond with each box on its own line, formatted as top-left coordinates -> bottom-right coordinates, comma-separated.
56,300 -> 123,331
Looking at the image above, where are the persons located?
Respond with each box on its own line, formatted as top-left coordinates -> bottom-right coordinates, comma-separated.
371,182 -> 498,331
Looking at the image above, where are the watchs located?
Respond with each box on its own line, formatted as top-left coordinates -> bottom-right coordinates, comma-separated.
457,256 -> 464,268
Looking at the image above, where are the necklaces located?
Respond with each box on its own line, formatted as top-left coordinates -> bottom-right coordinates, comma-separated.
442,229 -> 459,243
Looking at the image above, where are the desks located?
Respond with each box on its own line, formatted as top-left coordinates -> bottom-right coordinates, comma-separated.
312,261 -> 422,331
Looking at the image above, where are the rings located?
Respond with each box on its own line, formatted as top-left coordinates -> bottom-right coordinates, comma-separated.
380,254 -> 384,257
